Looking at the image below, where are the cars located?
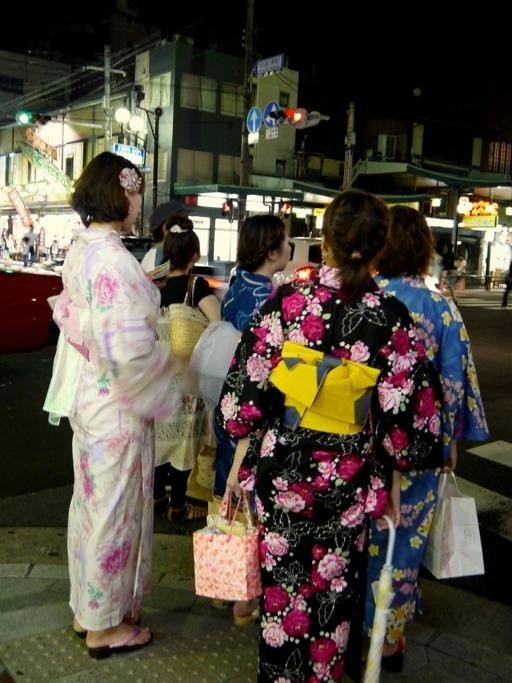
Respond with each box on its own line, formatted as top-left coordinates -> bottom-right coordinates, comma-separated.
1,260 -> 66,351
192,261 -> 238,300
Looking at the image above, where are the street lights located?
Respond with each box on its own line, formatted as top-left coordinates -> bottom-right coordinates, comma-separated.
114,108 -> 149,238
451,196 -> 472,256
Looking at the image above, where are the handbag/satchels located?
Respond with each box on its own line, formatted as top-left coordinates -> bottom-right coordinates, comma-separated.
194,496 -> 265,603
171,303 -> 209,359
424,486 -> 488,581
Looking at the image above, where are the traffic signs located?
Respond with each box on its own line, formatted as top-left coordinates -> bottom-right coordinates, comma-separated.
255,53 -> 285,74
113,143 -> 146,166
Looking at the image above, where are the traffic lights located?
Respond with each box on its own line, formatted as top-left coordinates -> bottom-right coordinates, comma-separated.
15,110 -> 52,125
269,107 -> 308,123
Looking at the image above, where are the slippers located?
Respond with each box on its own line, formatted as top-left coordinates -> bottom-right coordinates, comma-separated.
88,626 -> 153,659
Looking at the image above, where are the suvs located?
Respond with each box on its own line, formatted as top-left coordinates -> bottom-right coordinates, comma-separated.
229,237 -> 314,280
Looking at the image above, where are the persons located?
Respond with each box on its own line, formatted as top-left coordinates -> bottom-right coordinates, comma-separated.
1,224 -> 58,267
213,188 -> 442,680
139,202 -> 222,525
501,260 -> 512,308
428,250 -> 467,292
42,152 -> 194,658
